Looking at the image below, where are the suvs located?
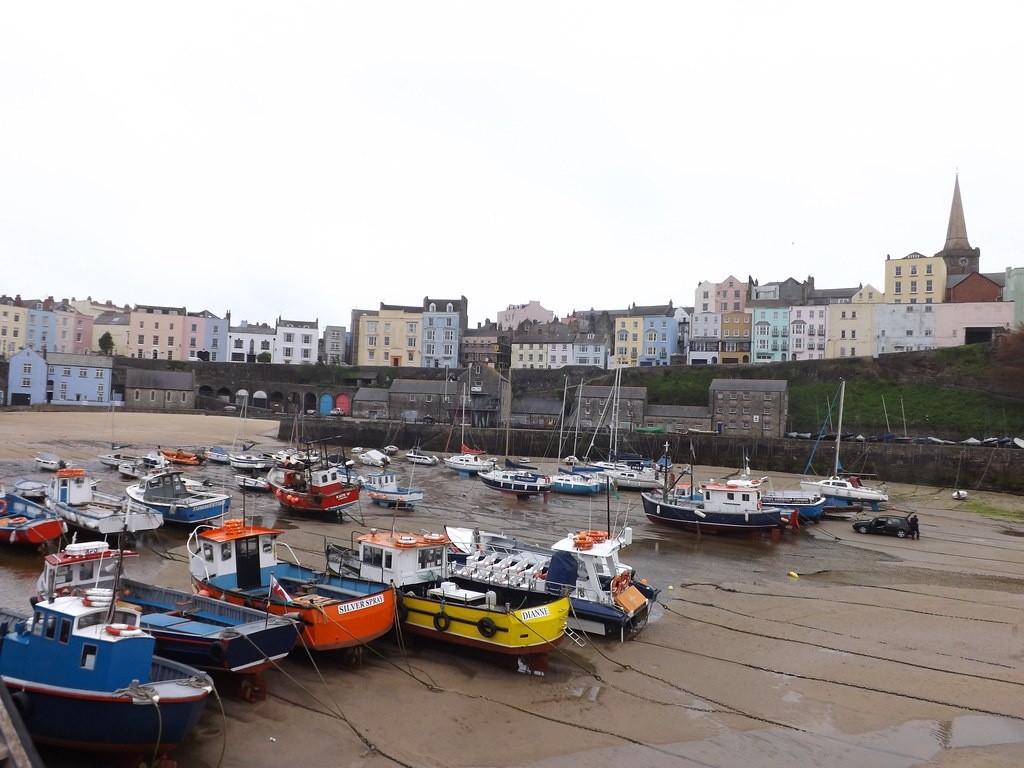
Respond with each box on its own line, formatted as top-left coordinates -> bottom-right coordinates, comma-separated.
853,509 -> 917,538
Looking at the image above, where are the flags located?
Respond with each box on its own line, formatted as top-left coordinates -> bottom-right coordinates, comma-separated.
272,576 -> 293,603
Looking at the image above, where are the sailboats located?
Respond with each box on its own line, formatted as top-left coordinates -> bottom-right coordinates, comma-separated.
271,391 -> 324,469
981,407 -> 1013,449
229,387 -> 277,471
786,392 -> 914,444
477,353 -> 664,499
443,382 -> 495,477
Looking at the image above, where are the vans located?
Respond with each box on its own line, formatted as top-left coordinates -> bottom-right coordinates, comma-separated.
189,356 -> 202,361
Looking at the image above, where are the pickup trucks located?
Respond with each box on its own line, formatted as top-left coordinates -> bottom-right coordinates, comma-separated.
330,408 -> 345,416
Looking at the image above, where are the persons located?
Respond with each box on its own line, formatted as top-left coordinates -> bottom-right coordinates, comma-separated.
910,515 -> 919,540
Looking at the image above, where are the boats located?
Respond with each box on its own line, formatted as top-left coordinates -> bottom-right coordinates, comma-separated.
44,466 -> 165,548
0,485 -> 64,550
159,449 -> 205,465
444,525 -> 662,641
916,437 -> 981,446
199,448 -> 232,462
98,452 -> 170,477
952,490 -> 968,500
1013,437 -> 1024,449
640,438 -> 781,541
186,517 -> 397,668
268,462 -> 362,512
359,449 -> 390,468
351,447 -> 362,453
406,447 -> 440,466
797,380 -> 888,511
34,456 -> 71,470
385,444 -> 399,454
234,475 -> 269,491
14,476 -> 98,502
656,476 -> 826,524
519,457 -> 530,463
320,453 -> 355,469
562,456 -> 579,464
30,537 -> 303,704
364,468 -> 422,508
687,428 -> 719,434
1,595 -> 214,768
320,528 -> 587,671
125,467 -> 229,525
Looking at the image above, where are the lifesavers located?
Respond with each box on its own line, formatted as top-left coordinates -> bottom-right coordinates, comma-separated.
395,537 -> 419,548
83,587 -> 113,609
573,536 -> 593,551
752,480 -> 759,484
433,612 -> 450,631
477,617 -> 496,639
396,589 -> 408,624
11,516 -> 26,524
0,499 -> 6,513
619,575 -> 629,590
581,529 -> 607,543
612,577 -> 621,597
105,622 -> 144,637
424,533 -> 446,544
222,519 -> 244,534
